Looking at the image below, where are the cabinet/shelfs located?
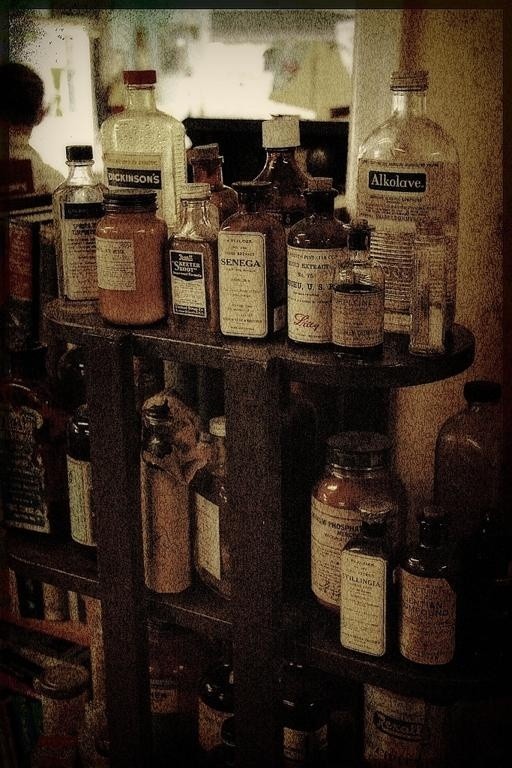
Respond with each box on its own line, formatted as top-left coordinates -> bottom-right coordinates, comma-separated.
0,289 -> 512,767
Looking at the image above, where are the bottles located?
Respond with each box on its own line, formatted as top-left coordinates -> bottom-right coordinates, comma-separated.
5,566 -> 504,768
46,67 -> 466,357
10,325 -> 507,671
